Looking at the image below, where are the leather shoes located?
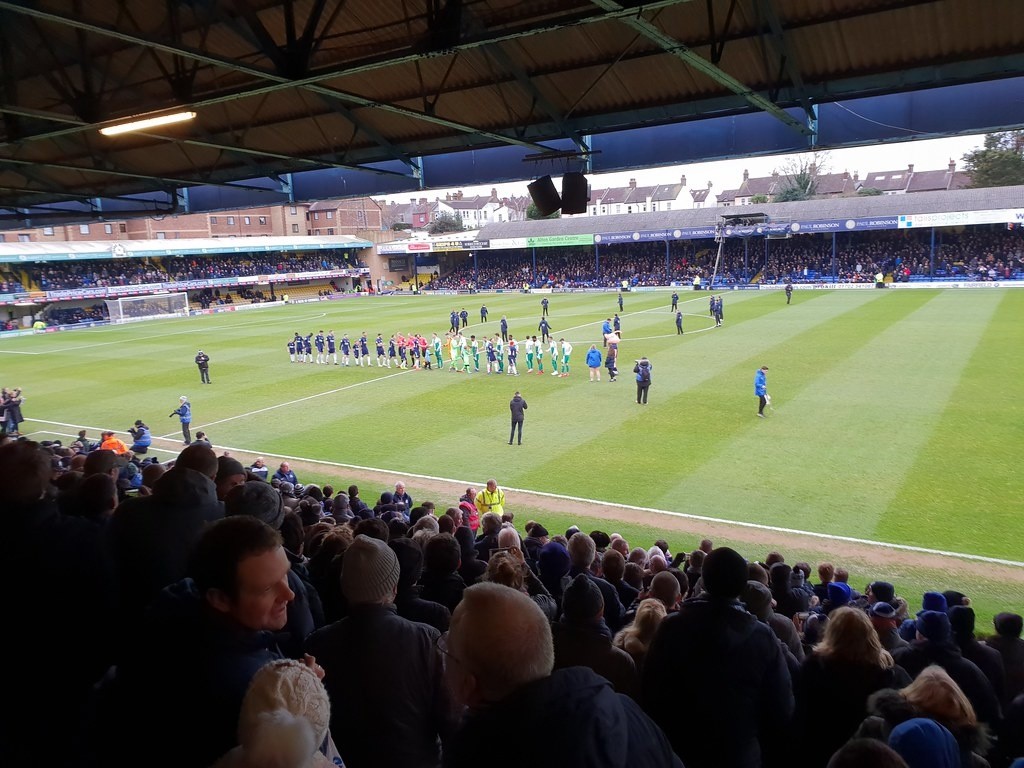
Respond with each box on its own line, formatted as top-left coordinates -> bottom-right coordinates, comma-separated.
508,442 -> 521,445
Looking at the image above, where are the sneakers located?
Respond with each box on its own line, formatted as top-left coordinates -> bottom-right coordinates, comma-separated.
290,356 -> 619,383
201,380 -> 211,383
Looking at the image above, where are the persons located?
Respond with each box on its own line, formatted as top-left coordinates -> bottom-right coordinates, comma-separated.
0,249 -> 369,294
480,304 -> 487,324
459,308 -> 468,327
538,317 -> 552,343
33,301 -> 108,331
387,332 -> 443,370
286,333 -> 313,363
449,309 -> 460,335
671,290 -> 678,312
506,334 -> 520,377
499,315 -> 508,342
507,391 -> 528,446
786,282 -> 793,305
441,332 -> 479,373
175,396 -> 191,446
352,332 -> 374,367
541,297 -> 550,316
192,286 -> 288,309
532,335 -> 544,375
0,421 -> 1023,768
314,330 -> 326,364
396,222 -> 1024,292
755,366 -> 768,418
480,333 -> 504,374
558,338 -> 573,377
709,294 -> 723,327
195,350 -> 211,384
375,334 -> 387,367
0,320 -> 20,332
617,294 -> 623,311
585,344 -> 602,382
602,319 -> 612,349
675,309 -> 684,335
525,335 -> 534,373
614,314 -> 621,339
633,357 -> 651,405
605,344 -> 618,382
319,289 -> 332,296
325,330 -> 338,365
546,337 -> 559,375
1,388 -> 23,436
339,333 -> 352,367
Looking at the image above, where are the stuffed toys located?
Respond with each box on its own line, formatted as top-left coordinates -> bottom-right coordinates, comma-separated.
604,330 -> 622,375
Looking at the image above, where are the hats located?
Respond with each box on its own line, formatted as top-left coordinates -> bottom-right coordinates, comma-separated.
237,660 -> 331,768
13,429 -> 286,548
135,420 -> 142,425
287,469 -> 1024,768
179,396 -> 187,401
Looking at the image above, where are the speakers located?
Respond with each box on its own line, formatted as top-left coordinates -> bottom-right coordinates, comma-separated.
560,172 -> 587,214
527,175 -> 561,216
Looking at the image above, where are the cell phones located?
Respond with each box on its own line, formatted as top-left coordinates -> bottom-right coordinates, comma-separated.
489,547 -> 514,558
